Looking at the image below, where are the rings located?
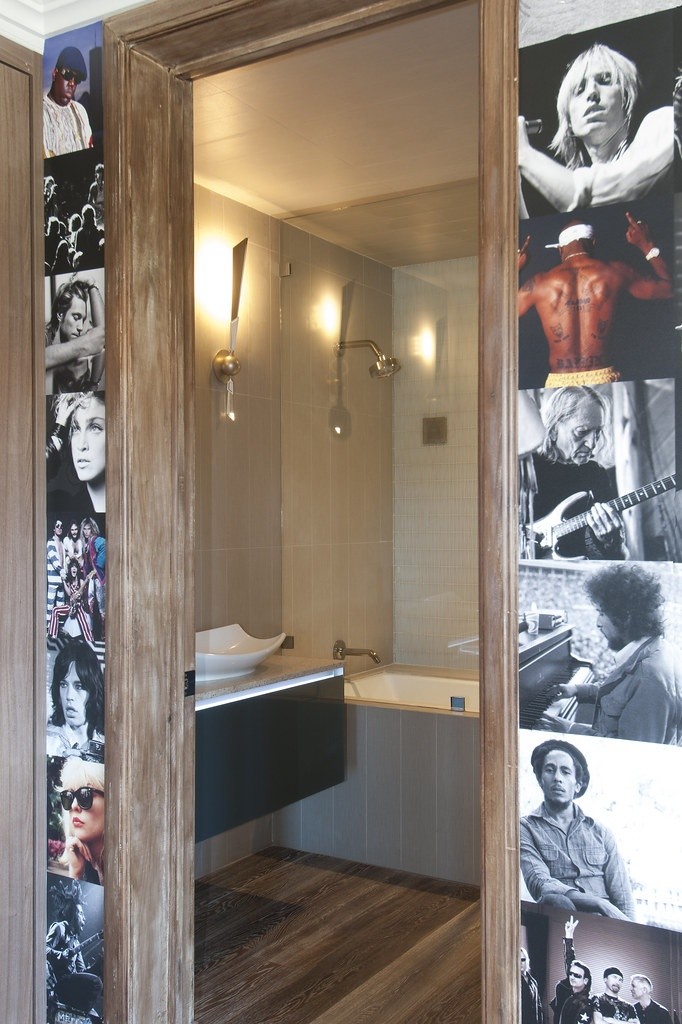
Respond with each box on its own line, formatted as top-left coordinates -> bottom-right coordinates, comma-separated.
518,249 -> 520,252
636,220 -> 642,223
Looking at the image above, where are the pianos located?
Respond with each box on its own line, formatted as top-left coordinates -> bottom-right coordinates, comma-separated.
517,622 -> 600,734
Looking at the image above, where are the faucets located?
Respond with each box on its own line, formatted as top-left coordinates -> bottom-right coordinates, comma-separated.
333,640 -> 383,664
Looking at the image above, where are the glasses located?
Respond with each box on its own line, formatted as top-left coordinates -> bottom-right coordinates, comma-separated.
59,785 -> 105,809
568,971 -> 582,978
520,958 -> 525,961
60,68 -> 82,84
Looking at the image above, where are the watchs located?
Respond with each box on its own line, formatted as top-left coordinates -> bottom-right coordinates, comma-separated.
646,247 -> 661,261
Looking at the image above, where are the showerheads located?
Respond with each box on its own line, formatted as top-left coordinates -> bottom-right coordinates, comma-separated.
333,340 -> 401,379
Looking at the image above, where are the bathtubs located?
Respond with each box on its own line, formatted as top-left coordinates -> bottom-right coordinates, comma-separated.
273,663 -> 480,886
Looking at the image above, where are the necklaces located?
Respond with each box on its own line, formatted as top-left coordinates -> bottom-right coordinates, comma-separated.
565,252 -> 590,260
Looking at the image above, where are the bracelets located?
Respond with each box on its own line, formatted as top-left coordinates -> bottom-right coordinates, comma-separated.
89,380 -> 100,386
88,284 -> 99,294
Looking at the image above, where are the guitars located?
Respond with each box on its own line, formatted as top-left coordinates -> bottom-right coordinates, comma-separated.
522,473 -> 680,562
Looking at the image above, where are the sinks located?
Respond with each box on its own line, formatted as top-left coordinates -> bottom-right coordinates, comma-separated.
195,623 -> 286,681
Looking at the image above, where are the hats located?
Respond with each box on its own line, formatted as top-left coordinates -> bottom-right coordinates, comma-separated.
531,740 -> 590,798
604,967 -> 623,978
56,46 -> 87,81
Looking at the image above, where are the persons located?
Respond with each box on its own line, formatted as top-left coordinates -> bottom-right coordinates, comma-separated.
47,516 -> 106,641
40,47 -> 94,159
533,563 -> 682,745
516,384 -> 634,559
59,756 -> 106,885
519,740 -> 636,922
46,880 -> 105,1024
519,916 -> 676,1024
43,274 -> 105,395
43,164 -> 106,277
48,392 -> 106,511
45,640 -> 105,760
515,43 -> 681,218
518,212 -> 674,389
